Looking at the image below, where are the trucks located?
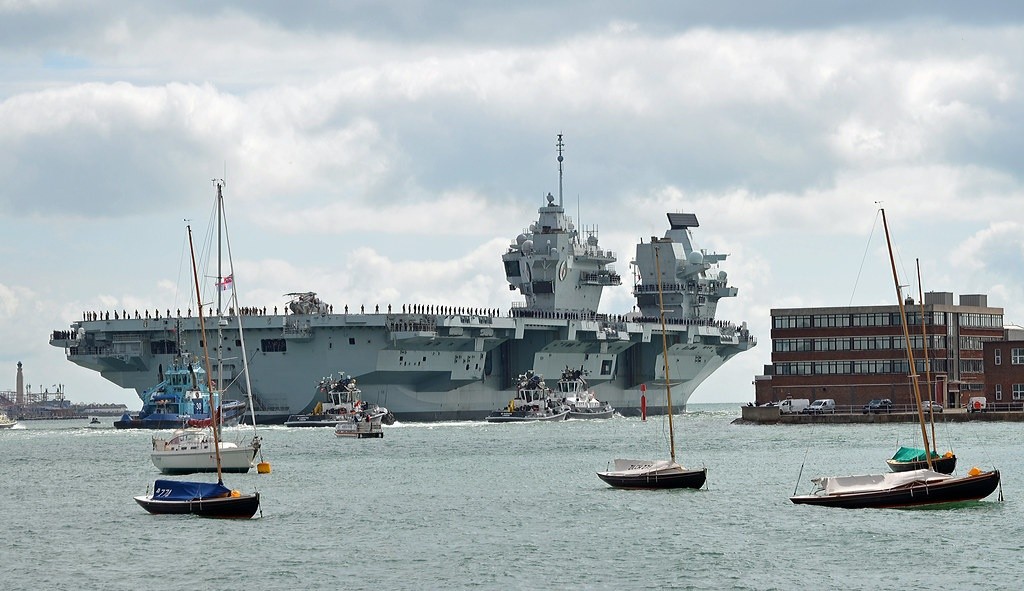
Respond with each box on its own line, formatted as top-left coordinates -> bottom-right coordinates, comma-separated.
775,399 -> 810,415
967,397 -> 987,412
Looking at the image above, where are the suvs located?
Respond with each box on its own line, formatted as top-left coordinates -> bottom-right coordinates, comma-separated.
863,399 -> 893,414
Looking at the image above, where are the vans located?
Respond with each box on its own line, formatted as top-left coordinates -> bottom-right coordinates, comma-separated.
808,399 -> 836,414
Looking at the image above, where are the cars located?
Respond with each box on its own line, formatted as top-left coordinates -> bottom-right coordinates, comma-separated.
917,401 -> 943,413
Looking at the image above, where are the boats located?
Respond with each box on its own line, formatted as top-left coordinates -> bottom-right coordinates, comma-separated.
133,226 -> 260,518
790,201 -> 1002,507
597,246 -> 708,489
486,370 -> 571,423
1,383 -> 89,427
151,178 -> 263,476
90,421 -> 101,423
335,391 -> 385,438
887,257 -> 958,475
549,365 -> 615,419
284,372 -> 388,428
115,320 -> 247,429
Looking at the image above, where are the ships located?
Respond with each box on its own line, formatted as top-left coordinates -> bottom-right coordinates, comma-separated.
48,133 -> 757,421
